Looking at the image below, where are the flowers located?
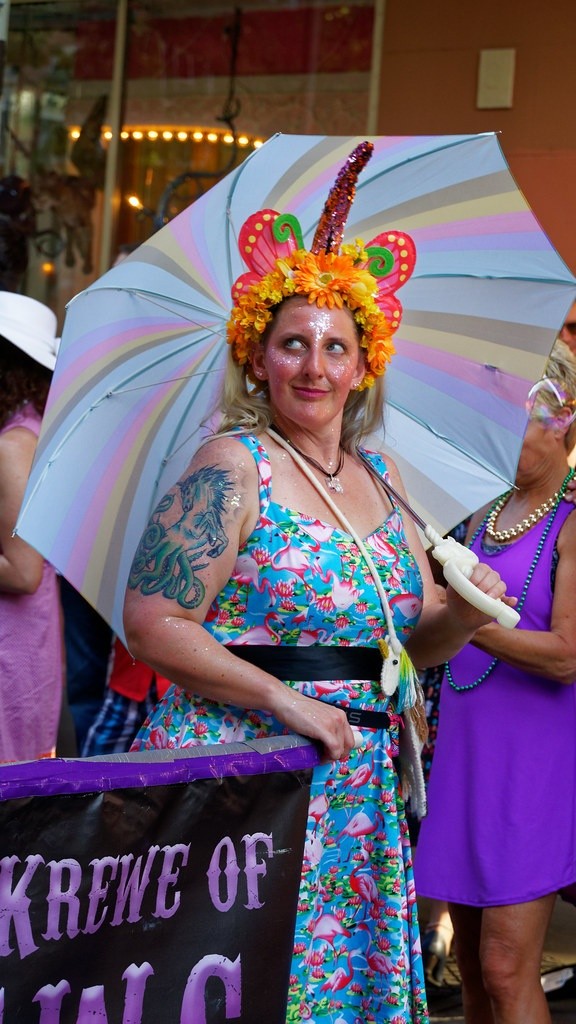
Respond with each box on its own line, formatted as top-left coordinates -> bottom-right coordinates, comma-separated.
224,237 -> 397,392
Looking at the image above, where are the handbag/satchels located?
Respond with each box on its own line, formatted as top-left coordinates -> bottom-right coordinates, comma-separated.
375,635 -> 428,822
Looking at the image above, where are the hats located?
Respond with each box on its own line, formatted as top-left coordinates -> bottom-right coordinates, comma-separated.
0,291 -> 61,372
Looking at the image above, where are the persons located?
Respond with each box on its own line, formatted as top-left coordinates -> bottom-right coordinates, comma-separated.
123,256 -> 518,1024
414,339 -> 576,1024
0,290 -> 62,764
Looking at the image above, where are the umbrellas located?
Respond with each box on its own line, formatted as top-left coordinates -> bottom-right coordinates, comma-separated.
14,132 -> 576,660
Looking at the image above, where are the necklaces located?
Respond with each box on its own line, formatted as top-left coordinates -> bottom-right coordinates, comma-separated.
268,423 -> 344,495
486,472 -> 576,542
445,466 -> 574,691
